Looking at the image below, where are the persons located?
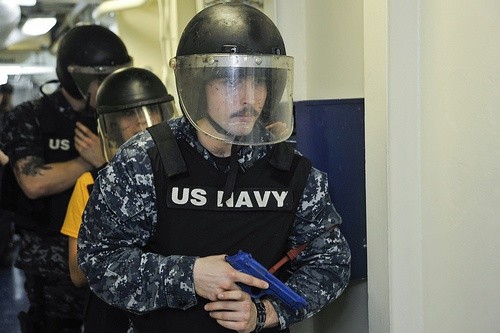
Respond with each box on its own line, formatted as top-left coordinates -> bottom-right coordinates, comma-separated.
61,67 -> 291,332
0,84 -> 14,118
76,2 -> 351,333
0,25 -> 133,332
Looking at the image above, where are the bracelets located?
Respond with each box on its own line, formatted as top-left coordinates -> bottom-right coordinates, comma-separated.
251,296 -> 266,333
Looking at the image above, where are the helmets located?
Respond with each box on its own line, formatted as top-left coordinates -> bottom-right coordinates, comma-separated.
96,66 -> 177,164
170,2 -> 297,145
54,24 -> 133,104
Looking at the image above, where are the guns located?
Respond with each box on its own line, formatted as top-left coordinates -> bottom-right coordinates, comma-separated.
225,250 -> 307,309
